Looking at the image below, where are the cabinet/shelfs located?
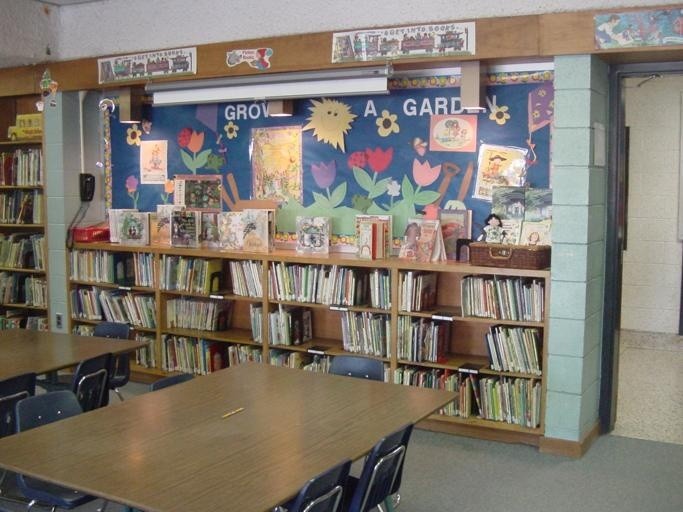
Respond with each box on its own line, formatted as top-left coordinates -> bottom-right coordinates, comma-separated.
65,238 -> 552,440
0,137 -> 49,330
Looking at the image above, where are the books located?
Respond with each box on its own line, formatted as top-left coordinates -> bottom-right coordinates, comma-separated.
1,141 -> 46,332
67,207 -> 543,435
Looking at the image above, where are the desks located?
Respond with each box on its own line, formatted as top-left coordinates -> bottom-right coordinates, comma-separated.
1,360 -> 461,510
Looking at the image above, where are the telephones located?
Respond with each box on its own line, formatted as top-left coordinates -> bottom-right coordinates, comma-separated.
80,174 -> 95,201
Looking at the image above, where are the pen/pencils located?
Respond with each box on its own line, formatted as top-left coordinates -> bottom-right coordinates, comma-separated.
221,407 -> 244,419
